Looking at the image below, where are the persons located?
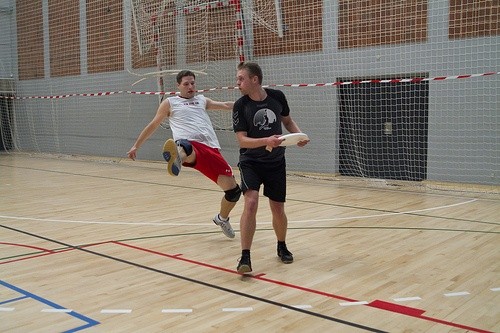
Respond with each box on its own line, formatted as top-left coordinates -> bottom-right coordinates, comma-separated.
232,62 -> 311,273
126,70 -> 242,239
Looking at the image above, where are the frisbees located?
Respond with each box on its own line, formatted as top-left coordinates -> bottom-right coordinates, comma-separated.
276,132 -> 309,147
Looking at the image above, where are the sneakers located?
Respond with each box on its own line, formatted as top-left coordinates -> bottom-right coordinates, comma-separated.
278,246 -> 293,262
213,214 -> 235,238
237,256 -> 252,272
163,139 -> 182,176
